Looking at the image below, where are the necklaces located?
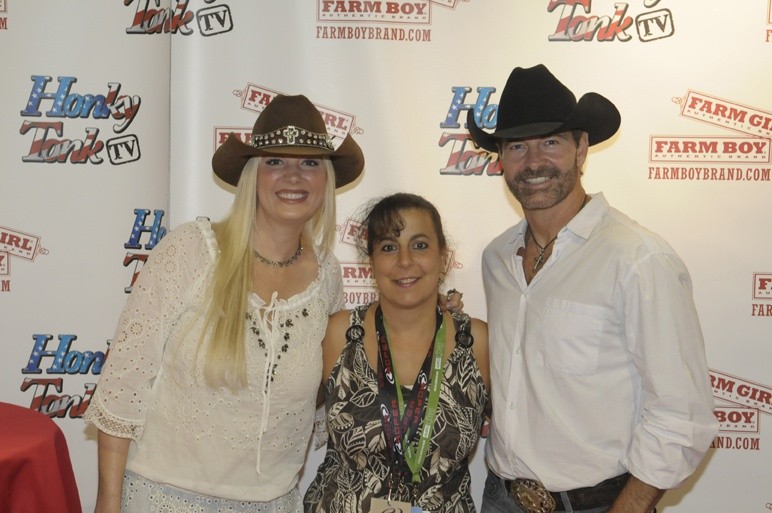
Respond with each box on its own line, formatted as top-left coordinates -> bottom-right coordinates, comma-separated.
524,193 -> 588,272
251,233 -> 304,269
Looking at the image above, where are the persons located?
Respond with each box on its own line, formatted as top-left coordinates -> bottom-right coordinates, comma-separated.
304,195 -> 488,513
467,64 -> 720,513
84,94 -> 365,513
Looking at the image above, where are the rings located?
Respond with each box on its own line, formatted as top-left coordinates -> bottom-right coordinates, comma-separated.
446,289 -> 463,300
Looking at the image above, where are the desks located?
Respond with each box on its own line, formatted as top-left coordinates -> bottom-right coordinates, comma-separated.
0,402 -> 82,513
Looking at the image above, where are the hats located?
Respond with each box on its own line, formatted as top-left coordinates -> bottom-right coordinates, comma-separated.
467,64 -> 620,153
212,94 -> 365,196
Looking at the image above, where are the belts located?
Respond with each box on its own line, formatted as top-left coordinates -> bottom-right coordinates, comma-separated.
489,470 -> 630,513
